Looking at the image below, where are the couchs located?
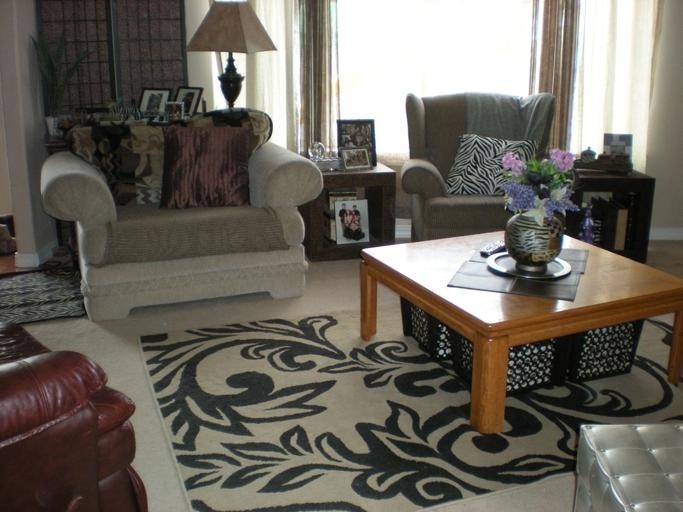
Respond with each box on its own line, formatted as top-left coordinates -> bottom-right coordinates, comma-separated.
0,322 -> 146,512
400,93 -> 555,241
41,150 -> 323,322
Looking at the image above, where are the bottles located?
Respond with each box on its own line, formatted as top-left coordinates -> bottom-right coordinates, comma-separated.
580,201 -> 594,245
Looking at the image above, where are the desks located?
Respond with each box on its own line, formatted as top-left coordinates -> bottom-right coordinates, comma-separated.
568,170 -> 655,264
305,162 -> 396,262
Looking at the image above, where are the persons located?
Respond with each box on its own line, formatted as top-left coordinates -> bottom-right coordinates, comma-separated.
339,203 -> 362,238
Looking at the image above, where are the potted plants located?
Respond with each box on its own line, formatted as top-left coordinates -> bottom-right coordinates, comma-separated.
30,29 -> 96,136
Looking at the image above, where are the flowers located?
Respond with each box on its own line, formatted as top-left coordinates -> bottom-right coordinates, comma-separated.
493,148 -> 581,220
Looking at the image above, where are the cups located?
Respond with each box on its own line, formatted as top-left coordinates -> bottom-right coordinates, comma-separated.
165,101 -> 185,121
581,146 -> 596,163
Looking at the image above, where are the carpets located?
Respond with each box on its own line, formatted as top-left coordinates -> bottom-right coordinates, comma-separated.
0,265 -> 85,324
138,302 -> 683,512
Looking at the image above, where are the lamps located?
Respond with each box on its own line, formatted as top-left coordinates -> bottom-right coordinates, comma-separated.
184,0 -> 278,107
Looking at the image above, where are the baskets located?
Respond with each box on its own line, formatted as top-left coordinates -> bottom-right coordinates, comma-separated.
400,297 -> 644,396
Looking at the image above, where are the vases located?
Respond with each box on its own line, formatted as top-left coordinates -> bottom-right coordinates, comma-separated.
504,214 -> 563,274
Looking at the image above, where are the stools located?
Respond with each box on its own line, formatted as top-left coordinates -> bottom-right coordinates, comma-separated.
573,424 -> 683,511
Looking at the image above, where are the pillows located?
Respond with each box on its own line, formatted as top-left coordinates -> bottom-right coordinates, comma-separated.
446,132 -> 540,195
64,110 -> 273,210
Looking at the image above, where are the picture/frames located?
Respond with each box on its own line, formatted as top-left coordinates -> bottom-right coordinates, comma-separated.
337,119 -> 377,165
341,147 -> 372,172
175,86 -> 204,117
139,88 -> 170,112
334,199 -> 370,244
166,102 -> 184,122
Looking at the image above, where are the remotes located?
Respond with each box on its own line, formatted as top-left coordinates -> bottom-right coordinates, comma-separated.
480,239 -> 507,257
